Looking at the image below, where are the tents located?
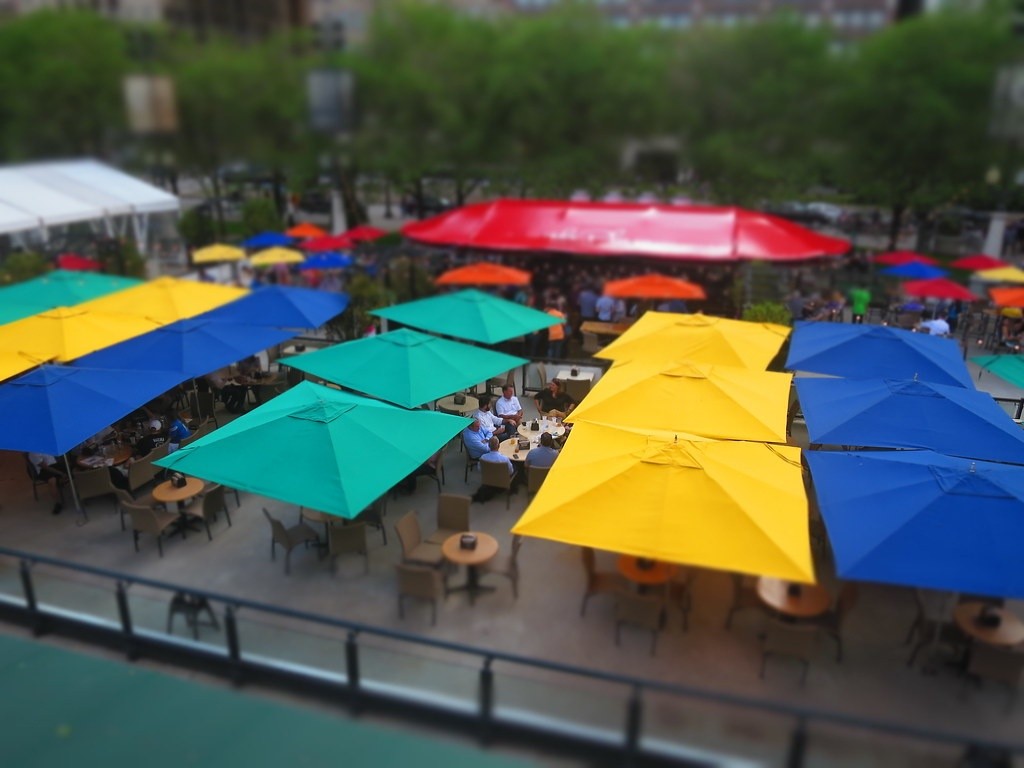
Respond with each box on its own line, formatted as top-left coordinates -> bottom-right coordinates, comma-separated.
0,153 -> 182,260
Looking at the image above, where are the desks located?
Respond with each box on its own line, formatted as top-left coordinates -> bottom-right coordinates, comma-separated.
436,395 -> 479,418
283,345 -> 319,355
442,531 -> 500,606
946,600 -> 1024,681
497,437 -> 540,461
753,575 -> 829,627
517,419 -> 565,442
76,440 -> 132,488
244,372 -> 278,404
615,554 -> 678,596
302,506 -> 343,556
557,370 -> 594,383
154,477 -> 205,529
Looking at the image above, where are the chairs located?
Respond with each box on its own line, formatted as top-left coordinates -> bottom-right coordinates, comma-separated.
902,584 -> 1002,699
395,362 -> 595,625
579,542 -> 692,655
729,566 -> 857,687
22,343 -> 388,574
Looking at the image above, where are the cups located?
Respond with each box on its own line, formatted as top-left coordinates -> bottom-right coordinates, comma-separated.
510,435 -> 516,444
514,444 -> 520,453
557,417 -> 562,426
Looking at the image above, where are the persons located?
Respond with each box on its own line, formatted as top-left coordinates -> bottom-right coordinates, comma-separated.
352,237 -> 728,501
1002,318 -> 1022,339
783,279 -> 873,322
24,352 -> 260,516
837,207 -> 883,233
495,383 -> 524,426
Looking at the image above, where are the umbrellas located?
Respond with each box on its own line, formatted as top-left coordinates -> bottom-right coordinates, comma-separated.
0,267 -> 353,422
794,371 -> 1024,464
513,416 -> 817,586
594,309 -> 793,370
561,356 -> 794,443
434,260 -> 532,285
975,265 -> 1024,284
785,318 -> 978,389
869,248 -> 939,265
0,363 -> 194,511
989,286 -> 1024,307
153,287 -> 567,569
602,275 -> 708,300
881,260 -> 951,279
950,254 -> 1010,270
900,278 -> 977,320
805,447 -> 1024,661
192,221 -> 389,284
